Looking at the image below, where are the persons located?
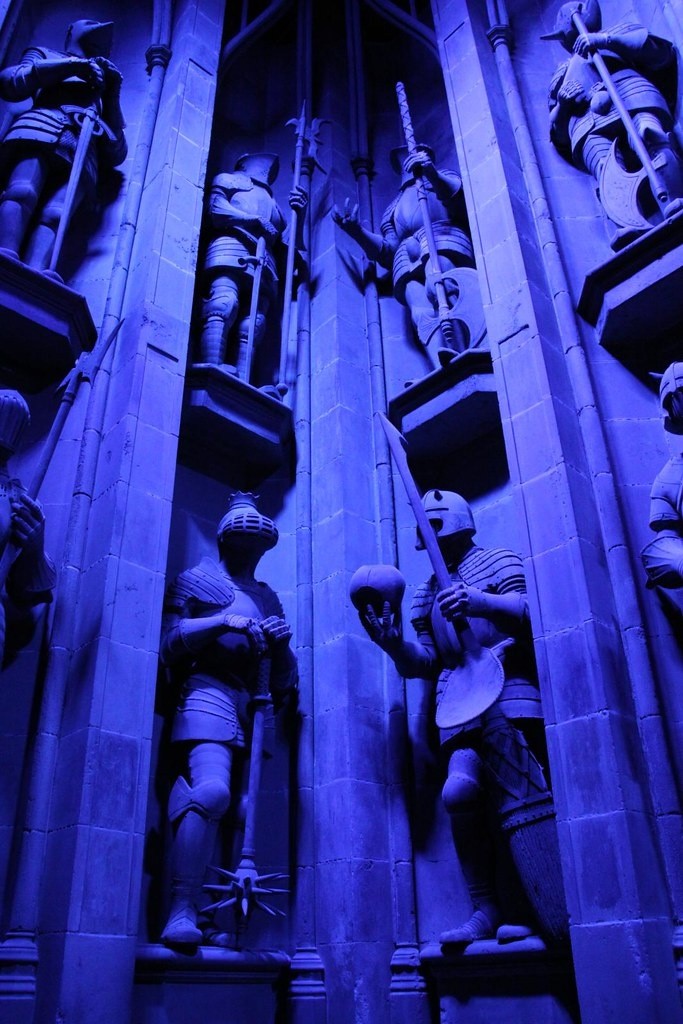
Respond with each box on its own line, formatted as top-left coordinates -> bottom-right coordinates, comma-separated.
2,0 -> 683,400
0,360 -> 683,946
